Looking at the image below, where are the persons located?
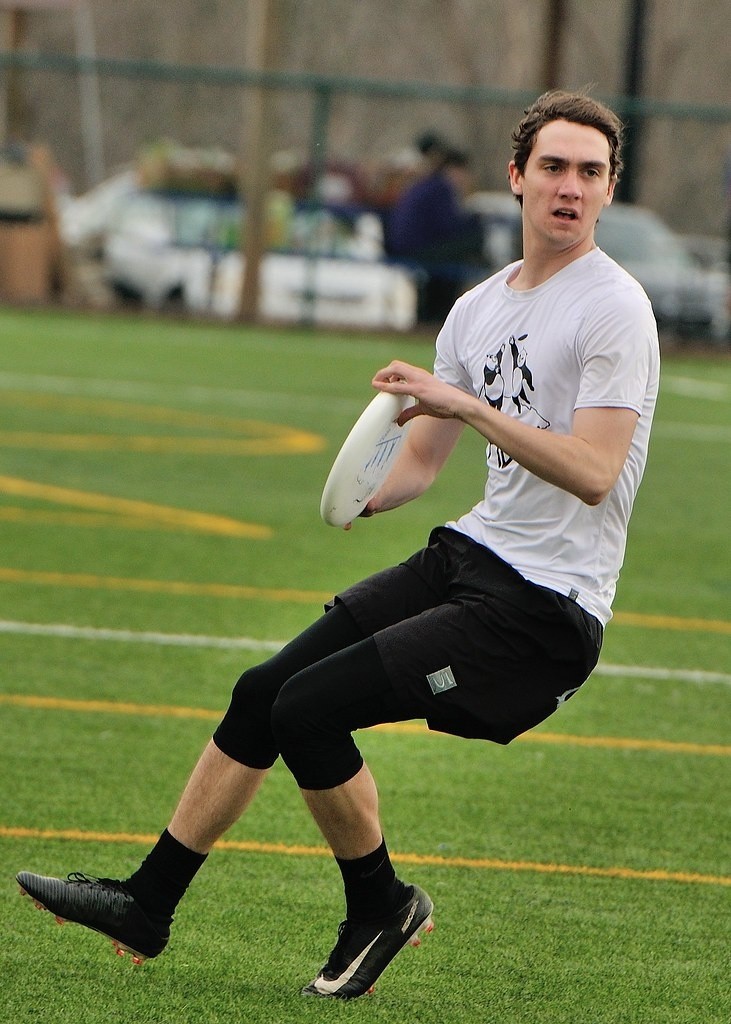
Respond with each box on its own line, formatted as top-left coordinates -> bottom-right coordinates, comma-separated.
389,133 -> 478,322
19,95 -> 662,993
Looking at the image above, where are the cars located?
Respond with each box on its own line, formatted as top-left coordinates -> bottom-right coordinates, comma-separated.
100,188 -> 415,335
458,188 -> 717,343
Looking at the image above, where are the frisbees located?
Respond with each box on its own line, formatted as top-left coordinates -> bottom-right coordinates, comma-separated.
320,390 -> 413,529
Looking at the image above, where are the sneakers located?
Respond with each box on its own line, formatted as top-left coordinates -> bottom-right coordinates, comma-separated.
299,884 -> 436,1000
14,871 -> 170,965
